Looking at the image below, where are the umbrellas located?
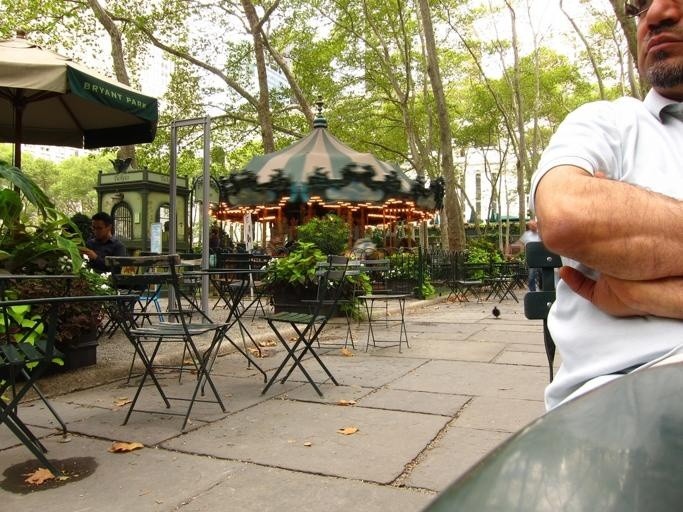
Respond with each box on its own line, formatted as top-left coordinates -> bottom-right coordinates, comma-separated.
0,27 -> 159,230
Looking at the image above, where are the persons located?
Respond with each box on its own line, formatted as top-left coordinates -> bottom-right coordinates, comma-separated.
81,211 -> 126,274
507,219 -> 543,292
525,1 -> 683,416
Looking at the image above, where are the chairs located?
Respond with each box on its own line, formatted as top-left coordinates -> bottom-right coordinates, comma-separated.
1,293 -> 141,476
259,255 -> 351,397
104,254 -> 232,431
1,240 -> 563,339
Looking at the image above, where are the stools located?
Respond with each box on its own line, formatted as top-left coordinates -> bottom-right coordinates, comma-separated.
357,293 -> 413,355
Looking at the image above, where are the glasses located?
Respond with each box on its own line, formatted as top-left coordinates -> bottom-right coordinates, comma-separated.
625,0 -> 653,18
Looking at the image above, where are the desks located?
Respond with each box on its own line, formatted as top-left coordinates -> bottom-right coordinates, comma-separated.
175,268 -> 275,396
421,362 -> 683,512
1,274 -> 79,443
